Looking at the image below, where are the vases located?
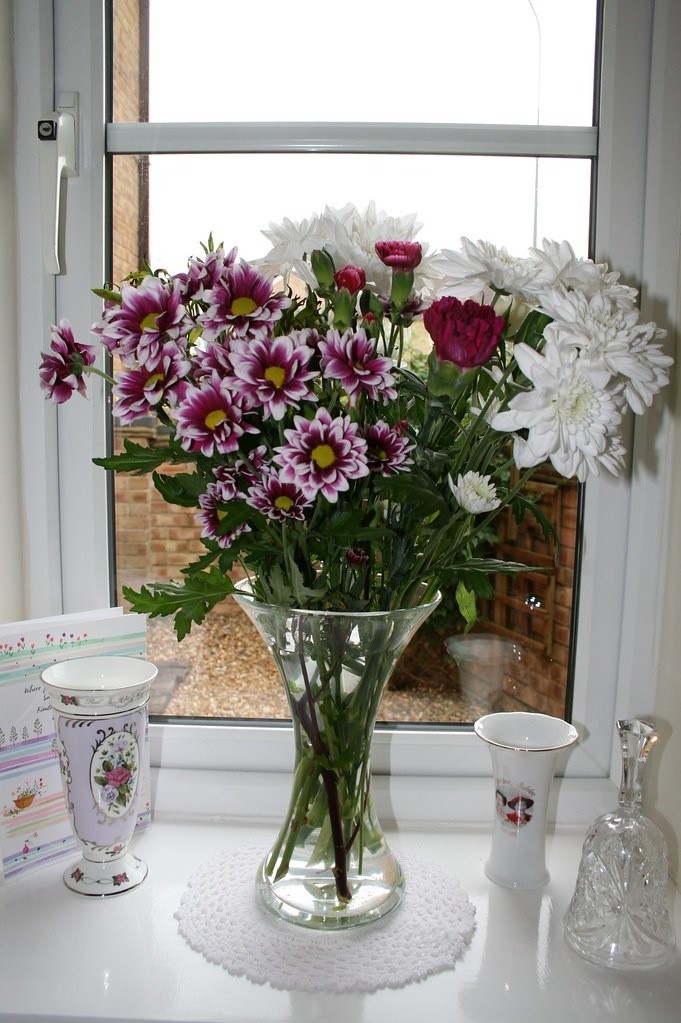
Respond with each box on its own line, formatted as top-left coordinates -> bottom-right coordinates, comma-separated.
233,571 -> 442,932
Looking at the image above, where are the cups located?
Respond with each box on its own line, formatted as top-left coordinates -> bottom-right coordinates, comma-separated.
474,712 -> 580,888
40,656 -> 158,897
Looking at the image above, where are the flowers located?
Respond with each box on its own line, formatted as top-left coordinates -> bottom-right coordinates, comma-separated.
38,201 -> 675,644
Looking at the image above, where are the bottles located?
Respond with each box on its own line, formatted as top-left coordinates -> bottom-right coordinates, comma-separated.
562,719 -> 677,970
